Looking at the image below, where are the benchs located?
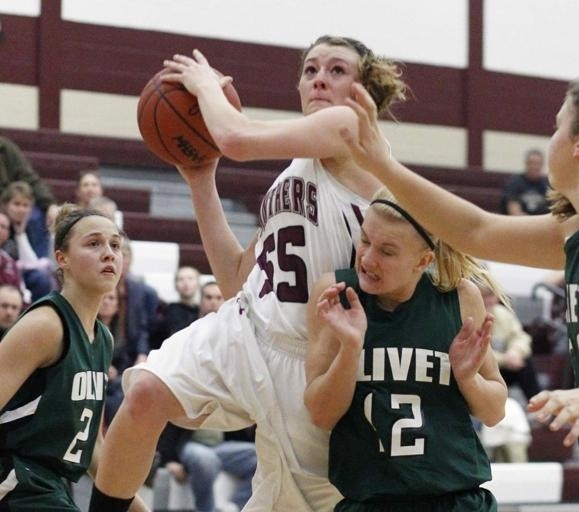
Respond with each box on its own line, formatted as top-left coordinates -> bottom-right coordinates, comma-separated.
1,148 -> 579,512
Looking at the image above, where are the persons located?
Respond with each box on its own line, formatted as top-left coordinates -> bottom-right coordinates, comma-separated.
302,186 -> 508,511
329,75 -> 578,460
503,149 -> 553,216
0,138 -> 256,512
471,275 -> 574,461
84,27 -> 410,512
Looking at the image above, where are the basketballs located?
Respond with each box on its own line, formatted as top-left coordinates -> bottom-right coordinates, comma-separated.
136,62 -> 243,170
